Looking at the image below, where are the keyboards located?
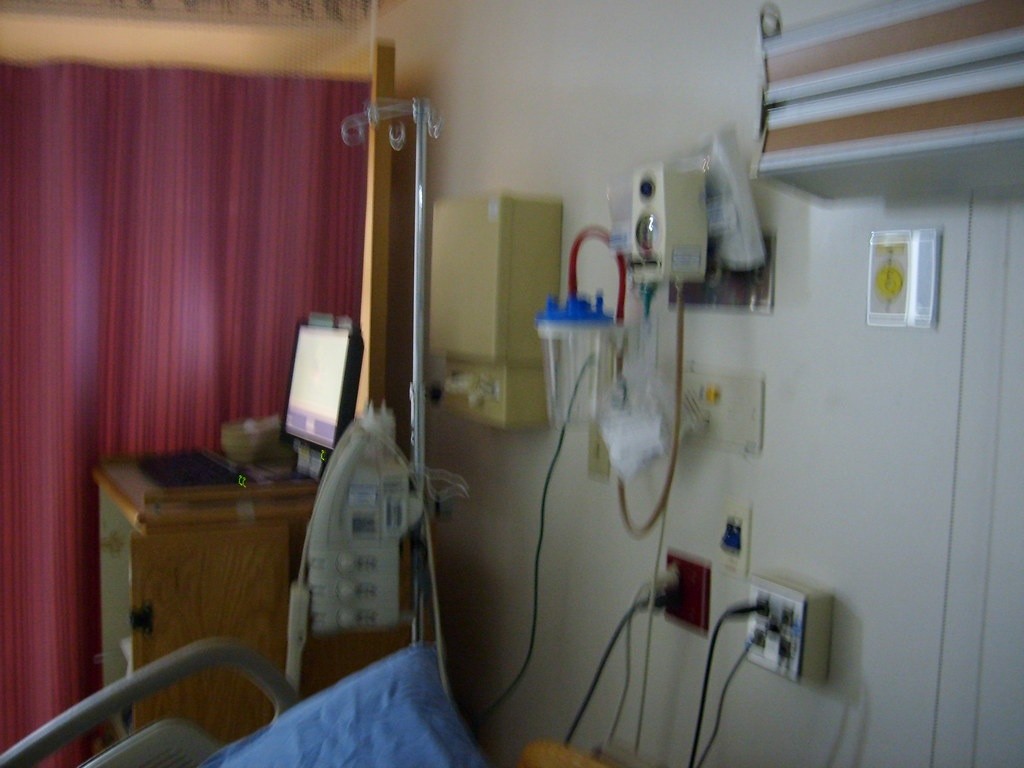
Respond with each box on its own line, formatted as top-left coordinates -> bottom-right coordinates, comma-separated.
138,446 -> 256,489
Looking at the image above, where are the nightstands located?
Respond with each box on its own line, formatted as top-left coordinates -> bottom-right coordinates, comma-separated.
92,464 -> 411,757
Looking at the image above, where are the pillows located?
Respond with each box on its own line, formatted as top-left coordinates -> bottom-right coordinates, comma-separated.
193,642 -> 485,768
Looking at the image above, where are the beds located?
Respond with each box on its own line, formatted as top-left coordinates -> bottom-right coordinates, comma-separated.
0,640 -> 601,768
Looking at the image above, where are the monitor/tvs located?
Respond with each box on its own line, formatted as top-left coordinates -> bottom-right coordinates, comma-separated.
280,319 -> 362,481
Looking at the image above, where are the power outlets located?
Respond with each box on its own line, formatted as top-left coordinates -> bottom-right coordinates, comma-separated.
659,550 -> 710,635
742,578 -> 831,684
